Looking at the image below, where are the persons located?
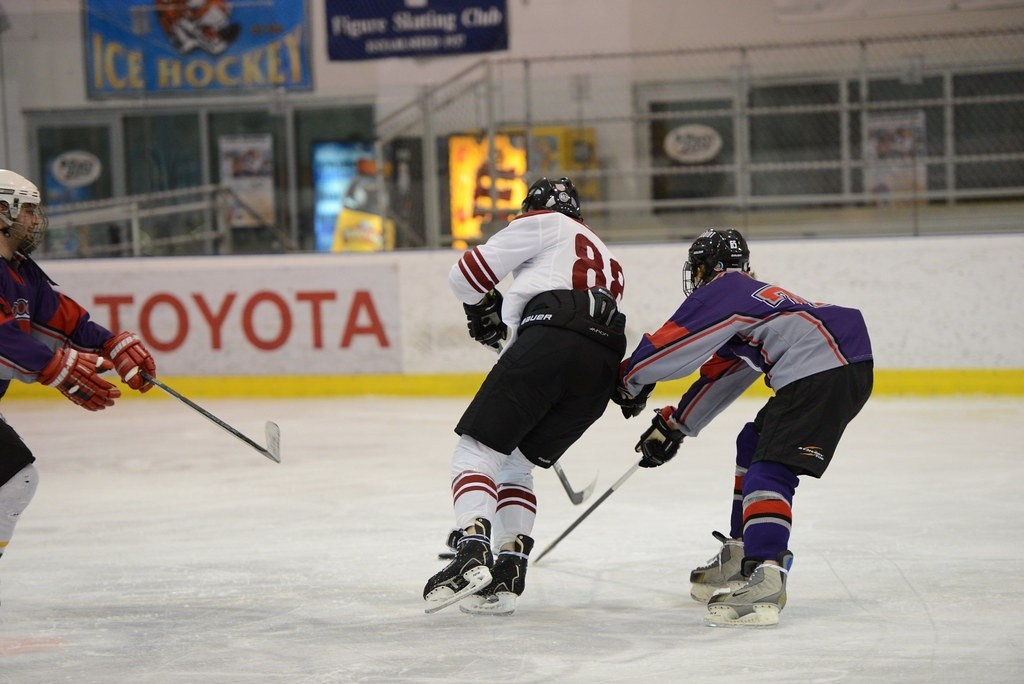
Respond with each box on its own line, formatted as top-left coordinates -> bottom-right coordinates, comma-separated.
423,177 -> 627,617
0,168 -> 156,557
610,228 -> 874,629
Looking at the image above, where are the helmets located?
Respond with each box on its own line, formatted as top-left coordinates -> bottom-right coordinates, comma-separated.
0,169 -> 42,237
522,176 -> 581,219
688,228 -> 750,283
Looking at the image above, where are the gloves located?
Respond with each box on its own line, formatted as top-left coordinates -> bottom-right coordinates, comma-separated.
635,406 -> 686,468
610,358 -> 656,420
35,347 -> 121,411
463,288 -> 512,355
99,331 -> 156,393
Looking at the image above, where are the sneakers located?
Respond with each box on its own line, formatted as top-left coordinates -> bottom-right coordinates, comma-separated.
459,534 -> 535,616
690,530 -> 793,626
423,517 -> 494,614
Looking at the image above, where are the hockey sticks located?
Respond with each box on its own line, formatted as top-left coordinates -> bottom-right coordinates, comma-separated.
436,437 -> 675,568
141,374 -> 282,464
484,315 -> 600,507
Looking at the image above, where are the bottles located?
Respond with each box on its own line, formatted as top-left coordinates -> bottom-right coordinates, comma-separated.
332,160 -> 395,251
472,151 -> 515,233
